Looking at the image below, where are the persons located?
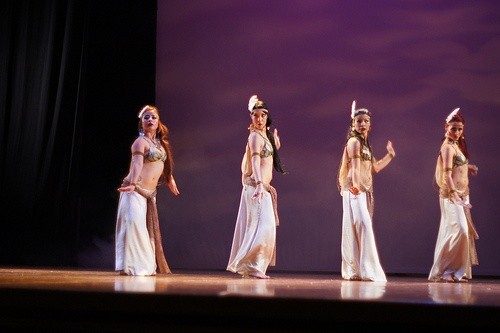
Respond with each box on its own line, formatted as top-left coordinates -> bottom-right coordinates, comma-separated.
113,275 -> 157,292
226,95 -> 283,279
338,100 -> 396,282
222,280 -> 275,297
427,108 -> 479,283
428,284 -> 474,304
341,281 -> 387,300
115,105 -> 180,275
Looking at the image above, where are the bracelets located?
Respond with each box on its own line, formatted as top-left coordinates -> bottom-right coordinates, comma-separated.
256,181 -> 262,185
130,183 -> 136,186
449,189 -> 456,193
